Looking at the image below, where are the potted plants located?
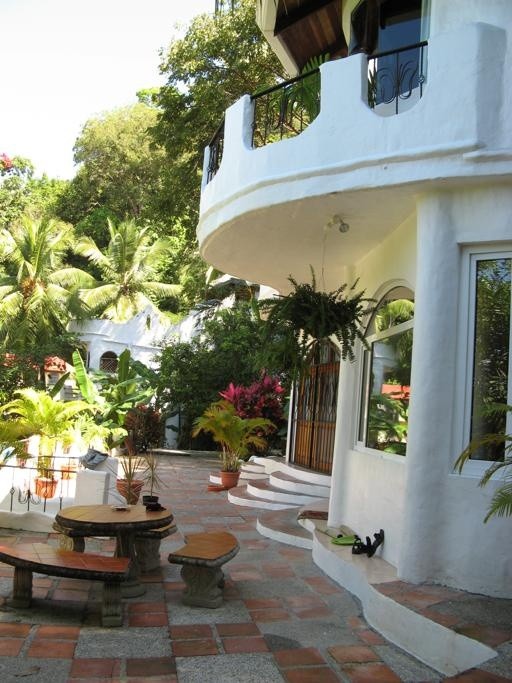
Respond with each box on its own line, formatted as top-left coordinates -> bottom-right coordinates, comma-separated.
1,387 -> 98,496
190,396 -> 278,489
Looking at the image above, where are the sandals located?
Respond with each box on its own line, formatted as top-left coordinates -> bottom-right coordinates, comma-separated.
351,538 -> 366,554
366,528 -> 385,559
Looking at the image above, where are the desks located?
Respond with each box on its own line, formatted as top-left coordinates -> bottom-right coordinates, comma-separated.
51,501 -> 173,598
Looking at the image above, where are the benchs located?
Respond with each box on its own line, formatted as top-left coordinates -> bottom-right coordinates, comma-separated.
165,526 -> 238,610
0,543 -> 135,628
